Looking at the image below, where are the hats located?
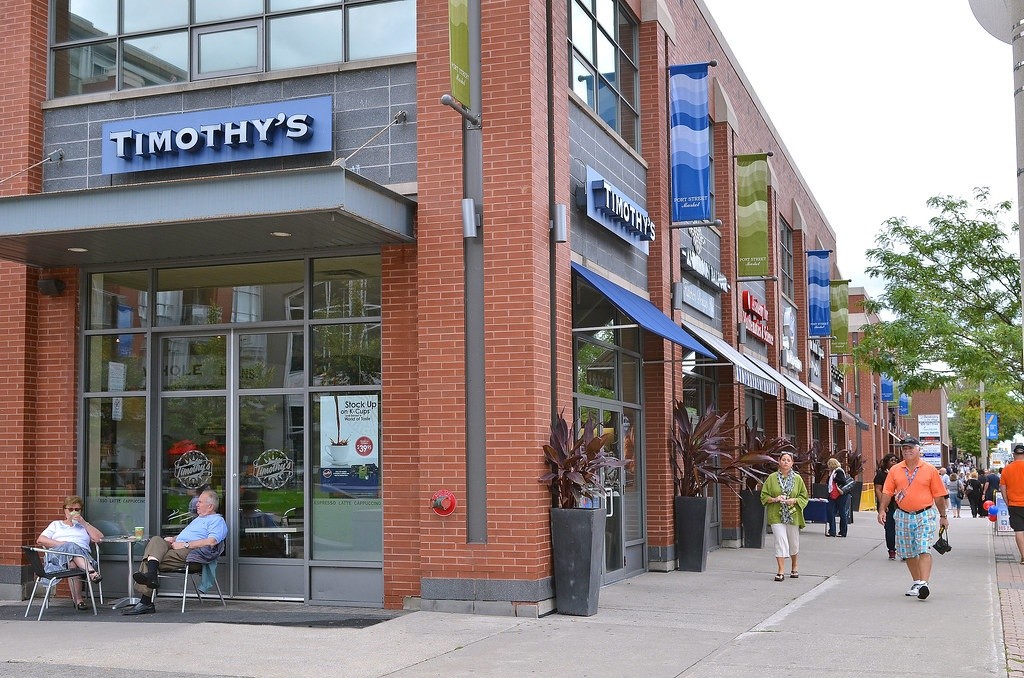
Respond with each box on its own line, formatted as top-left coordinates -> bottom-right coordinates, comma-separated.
1014,445 -> 1024,453
900,436 -> 920,444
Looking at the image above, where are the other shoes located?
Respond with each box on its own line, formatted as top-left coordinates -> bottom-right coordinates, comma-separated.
888,550 -> 896,560
74,601 -> 88,611
790,571 -> 799,578
91,573 -> 102,583
774,574 -> 785,581
837,534 -> 843,537
825,533 -> 835,537
1020,560 -> 1024,564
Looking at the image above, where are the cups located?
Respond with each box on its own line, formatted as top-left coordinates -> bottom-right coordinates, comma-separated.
71,511 -> 78,524
134,526 -> 144,541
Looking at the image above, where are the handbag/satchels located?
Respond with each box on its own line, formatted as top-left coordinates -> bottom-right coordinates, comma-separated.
957,491 -> 963,499
834,477 -> 855,495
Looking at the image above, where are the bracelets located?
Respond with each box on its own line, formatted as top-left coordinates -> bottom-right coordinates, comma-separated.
85,523 -> 89,527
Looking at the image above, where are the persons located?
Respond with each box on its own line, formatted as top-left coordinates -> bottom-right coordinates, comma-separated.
874,454 -> 900,560
939,457 -> 1003,518
242,492 -> 283,540
825,458 -> 850,537
36,496 -> 104,610
877,437 -> 948,599
179,484 -> 211,514
123,491 -> 228,614
761,451 -> 809,580
1000,445 -> 1024,565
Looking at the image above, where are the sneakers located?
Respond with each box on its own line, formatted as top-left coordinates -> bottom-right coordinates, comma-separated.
917,581 -> 930,599
905,584 -> 922,596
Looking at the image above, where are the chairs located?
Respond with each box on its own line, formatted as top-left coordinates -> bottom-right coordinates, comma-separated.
21,542 -> 104,622
151,540 -> 227,614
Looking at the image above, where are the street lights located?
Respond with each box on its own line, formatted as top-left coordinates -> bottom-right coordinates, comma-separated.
970,1 -> 1023,327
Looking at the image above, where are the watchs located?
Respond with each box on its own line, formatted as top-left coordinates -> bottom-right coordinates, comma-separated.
940,515 -> 947,519
185,542 -> 189,549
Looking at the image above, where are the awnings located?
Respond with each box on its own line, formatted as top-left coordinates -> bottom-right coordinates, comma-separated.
833,398 -> 868,430
889,431 -> 903,441
680,320 -> 777,397
812,389 -> 856,427
784,375 -> 838,419
571,261 -> 717,365
744,354 -> 813,410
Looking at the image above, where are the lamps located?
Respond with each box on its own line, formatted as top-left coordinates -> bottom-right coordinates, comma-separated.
808,368 -> 814,382
844,392 -> 852,404
872,413 -> 902,436
461,197 -> 480,238
737,323 -> 746,344
778,349 -> 788,367
670,282 -> 683,310
548,205 -> 568,243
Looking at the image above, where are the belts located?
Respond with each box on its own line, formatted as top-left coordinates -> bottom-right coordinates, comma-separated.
898,506 -> 932,515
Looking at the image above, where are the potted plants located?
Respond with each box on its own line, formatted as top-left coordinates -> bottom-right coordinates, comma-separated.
538,406 -> 633,617
669,399 -> 867,572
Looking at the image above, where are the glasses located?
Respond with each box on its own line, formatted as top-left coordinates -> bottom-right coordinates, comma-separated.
780,451 -> 793,460
890,459 -> 899,462
65,507 -> 82,512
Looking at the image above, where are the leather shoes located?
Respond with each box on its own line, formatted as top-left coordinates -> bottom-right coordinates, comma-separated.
133,571 -> 159,589
123,602 -> 156,615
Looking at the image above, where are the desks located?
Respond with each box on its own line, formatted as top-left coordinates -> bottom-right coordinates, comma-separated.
95,535 -> 152,610
803,498 -> 831,536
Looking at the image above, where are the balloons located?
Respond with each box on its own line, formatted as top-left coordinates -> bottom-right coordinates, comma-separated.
983,500 -> 998,522
